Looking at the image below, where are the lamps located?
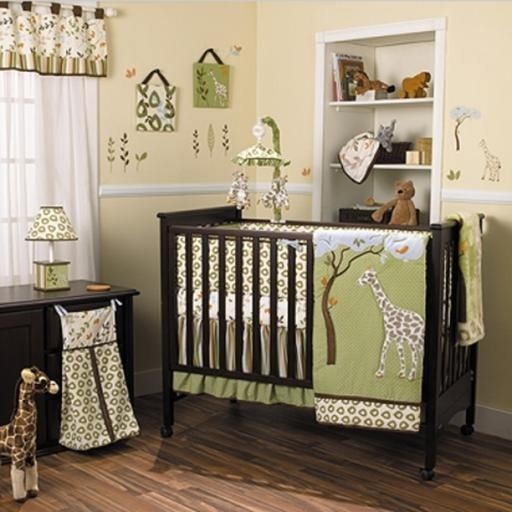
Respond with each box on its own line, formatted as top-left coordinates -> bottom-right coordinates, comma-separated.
25,206 -> 79,291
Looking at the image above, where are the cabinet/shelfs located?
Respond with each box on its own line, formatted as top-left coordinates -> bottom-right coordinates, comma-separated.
310,15 -> 447,226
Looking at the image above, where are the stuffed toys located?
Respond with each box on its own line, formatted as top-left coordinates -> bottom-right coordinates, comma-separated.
0,367 -> 60,503
372,181 -> 416,225
400,72 -> 431,98
353,70 -> 395,100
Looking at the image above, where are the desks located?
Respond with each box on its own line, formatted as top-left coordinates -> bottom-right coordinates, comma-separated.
1,279 -> 141,463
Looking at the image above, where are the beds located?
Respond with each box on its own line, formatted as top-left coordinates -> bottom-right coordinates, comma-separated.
157,209 -> 482,479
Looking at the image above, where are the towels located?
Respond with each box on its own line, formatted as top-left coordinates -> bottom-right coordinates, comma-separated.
445,212 -> 486,347
